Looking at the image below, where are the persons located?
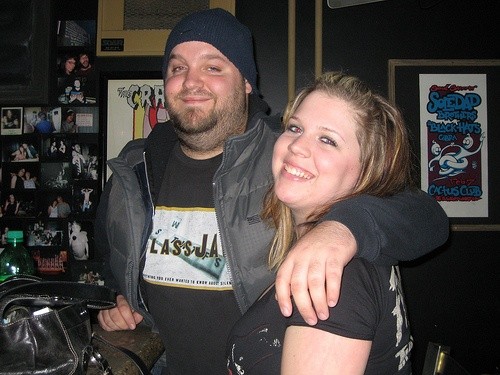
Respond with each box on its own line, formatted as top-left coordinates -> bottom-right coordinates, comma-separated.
72,145 -> 99,179
68,80 -> 86,105
10,140 -> 66,160
11,168 -> 68,189
226,71 -> 414,375
2,110 -> 19,129
49,201 -> 59,217
93,9 -> 450,375
68,220 -> 89,261
62,108 -> 78,133
57,197 -> 71,217
78,53 -> 95,102
35,111 -> 52,134
2,227 -> 9,246
56,56 -> 77,106
81,188 -> 93,211
28,223 -> 55,245
5,194 -> 20,217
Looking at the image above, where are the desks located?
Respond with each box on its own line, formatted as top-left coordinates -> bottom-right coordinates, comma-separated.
91,321 -> 167,374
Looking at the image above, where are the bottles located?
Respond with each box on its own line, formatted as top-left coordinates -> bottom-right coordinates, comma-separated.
0,231 -> 35,284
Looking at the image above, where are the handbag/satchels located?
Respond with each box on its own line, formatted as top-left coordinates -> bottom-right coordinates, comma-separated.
1,276 -> 117,375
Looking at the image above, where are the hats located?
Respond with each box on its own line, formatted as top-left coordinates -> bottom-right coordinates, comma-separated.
164,8 -> 256,95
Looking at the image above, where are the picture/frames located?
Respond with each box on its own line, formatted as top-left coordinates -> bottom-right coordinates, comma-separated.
386,58 -> 500,233
0,49 -> 170,249
